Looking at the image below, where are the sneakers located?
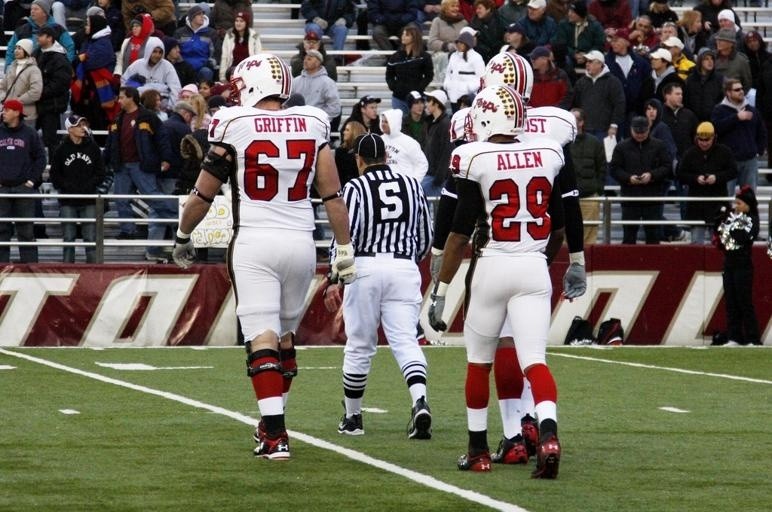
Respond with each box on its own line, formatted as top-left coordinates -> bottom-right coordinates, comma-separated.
458,454 -> 492,471
491,434 -> 526,463
254,438 -> 292,461
406,397 -> 434,439
251,425 -> 269,443
337,414 -> 366,435
531,436 -> 561,480
520,414 -> 539,455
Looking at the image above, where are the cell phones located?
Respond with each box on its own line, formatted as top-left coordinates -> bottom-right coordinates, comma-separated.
704,174 -> 709,180
635,177 -> 642,179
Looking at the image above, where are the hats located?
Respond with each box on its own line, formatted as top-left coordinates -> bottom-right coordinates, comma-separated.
235,10 -> 252,26
15,37 -> 34,57
407,90 -> 422,108
424,86 -> 447,107
63,114 -> 88,129
3,99 -> 28,118
77,5 -> 225,114
31,1 -> 51,18
455,25 -> 479,49
34,25 -> 56,38
360,94 -> 381,106
303,31 -> 325,63
351,130 -> 387,165
528,1 -> 764,140
502,22 -> 526,35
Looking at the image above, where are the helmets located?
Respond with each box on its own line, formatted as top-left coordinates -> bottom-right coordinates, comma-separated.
483,51 -> 535,101
224,54 -> 295,107
471,84 -> 528,142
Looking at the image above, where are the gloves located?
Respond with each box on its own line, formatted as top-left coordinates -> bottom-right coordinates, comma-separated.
428,282 -> 449,333
334,244 -> 356,284
171,231 -> 197,271
562,252 -> 588,301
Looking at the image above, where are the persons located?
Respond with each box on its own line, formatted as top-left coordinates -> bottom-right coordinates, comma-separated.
322,132 -> 435,442
0,2 -> 772,267
713,183 -> 764,347
428,49 -> 578,468
170,52 -> 356,465
429,82 -> 590,481
